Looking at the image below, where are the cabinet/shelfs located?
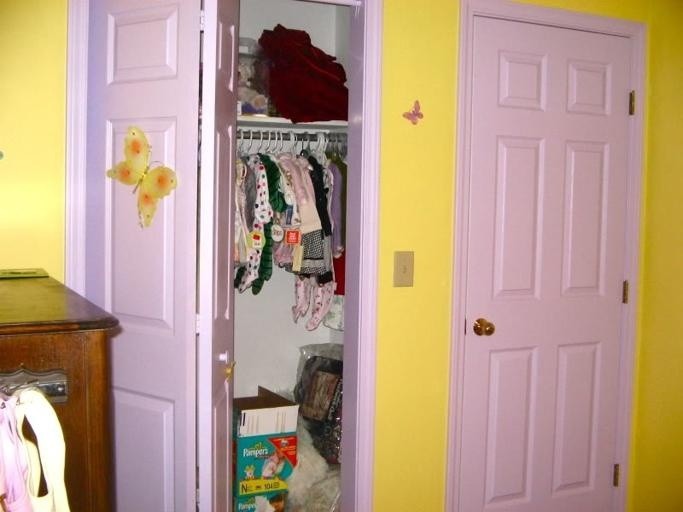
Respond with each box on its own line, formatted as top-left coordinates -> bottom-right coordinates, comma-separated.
0,267 -> 121,512
65,1 -> 384,512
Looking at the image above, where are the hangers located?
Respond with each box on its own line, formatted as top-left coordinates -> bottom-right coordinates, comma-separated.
237,128 -> 348,160
